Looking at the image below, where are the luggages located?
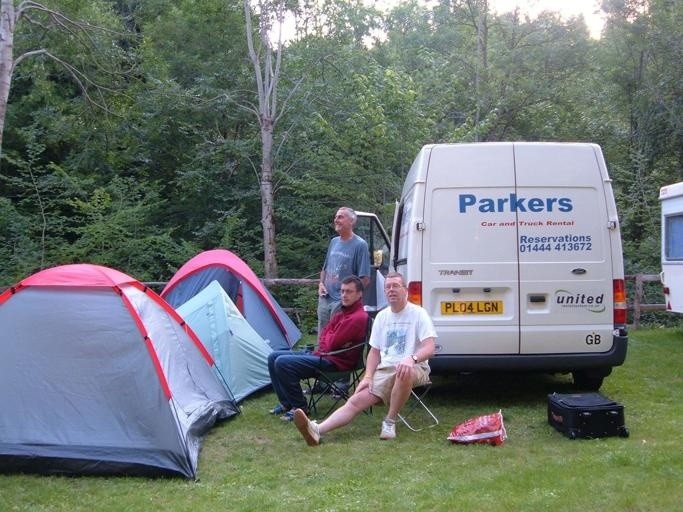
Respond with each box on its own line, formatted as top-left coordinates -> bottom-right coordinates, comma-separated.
545,390 -> 630,440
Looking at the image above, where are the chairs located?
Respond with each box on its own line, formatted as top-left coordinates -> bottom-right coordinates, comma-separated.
297,315 -> 378,423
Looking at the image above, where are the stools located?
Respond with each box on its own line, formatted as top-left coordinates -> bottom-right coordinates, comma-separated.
386,379 -> 438,433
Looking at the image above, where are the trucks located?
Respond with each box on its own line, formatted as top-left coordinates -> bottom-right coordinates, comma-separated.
350,140 -> 628,392
656,181 -> 683,316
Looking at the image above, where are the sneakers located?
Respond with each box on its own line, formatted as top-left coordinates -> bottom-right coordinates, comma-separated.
293,409 -> 319,446
379,419 -> 397,442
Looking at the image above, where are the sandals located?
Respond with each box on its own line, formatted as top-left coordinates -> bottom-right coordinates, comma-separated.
303,385 -> 348,399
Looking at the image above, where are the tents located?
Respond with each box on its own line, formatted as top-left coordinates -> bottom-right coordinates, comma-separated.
0,263 -> 240,483
158,249 -> 302,352
169,281 -> 276,406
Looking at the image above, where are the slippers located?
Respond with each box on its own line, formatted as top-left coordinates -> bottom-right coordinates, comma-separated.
271,405 -> 309,421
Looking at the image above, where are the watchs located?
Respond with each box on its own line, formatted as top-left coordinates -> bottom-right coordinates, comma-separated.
409,355 -> 418,364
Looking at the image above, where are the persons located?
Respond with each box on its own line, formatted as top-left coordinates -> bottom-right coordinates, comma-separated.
293,271 -> 438,448
301,206 -> 370,400
266,275 -> 369,423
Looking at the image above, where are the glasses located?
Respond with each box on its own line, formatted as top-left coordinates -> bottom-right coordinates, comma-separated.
338,289 -> 362,295
384,283 -> 405,291
